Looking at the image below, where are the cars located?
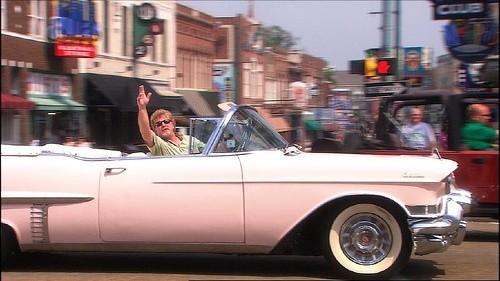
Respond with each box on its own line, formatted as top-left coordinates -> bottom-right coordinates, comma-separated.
372,88 -> 500,215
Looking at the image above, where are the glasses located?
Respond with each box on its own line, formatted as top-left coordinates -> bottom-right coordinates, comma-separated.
153,118 -> 172,129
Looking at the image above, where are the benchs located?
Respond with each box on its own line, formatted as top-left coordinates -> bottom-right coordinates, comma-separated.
386,132 -> 401,149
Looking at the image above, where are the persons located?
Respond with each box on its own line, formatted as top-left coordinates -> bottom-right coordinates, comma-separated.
137,85 -> 242,155
63,135 -> 90,147
461,104 -> 500,150
398,108 -> 436,149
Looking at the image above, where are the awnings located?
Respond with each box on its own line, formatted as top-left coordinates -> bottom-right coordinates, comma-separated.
304,121 -> 323,129
267,117 -> 290,132
322,124 -> 339,131
1,93 -> 35,109
178,88 -> 225,118
29,95 -> 86,110
86,72 -> 173,112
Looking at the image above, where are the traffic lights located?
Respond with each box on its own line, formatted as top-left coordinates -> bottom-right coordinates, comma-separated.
377,58 -> 400,76
348,59 -> 379,80
149,18 -> 165,35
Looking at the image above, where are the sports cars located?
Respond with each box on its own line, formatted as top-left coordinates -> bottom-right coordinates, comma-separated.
1,105 -> 476,281
